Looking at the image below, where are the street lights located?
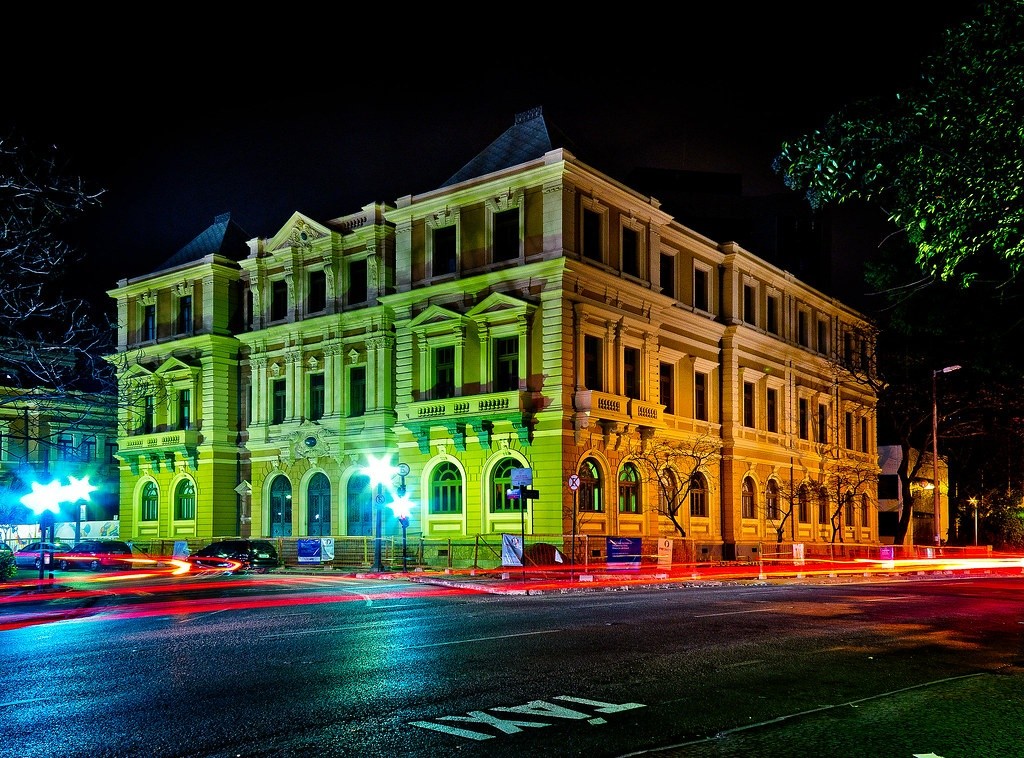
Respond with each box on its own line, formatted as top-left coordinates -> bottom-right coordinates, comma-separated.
389,490 -> 416,573
930,364 -> 961,560
363,454 -> 396,573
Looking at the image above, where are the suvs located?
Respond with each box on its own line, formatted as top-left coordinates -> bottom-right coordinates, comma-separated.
184,540 -> 278,574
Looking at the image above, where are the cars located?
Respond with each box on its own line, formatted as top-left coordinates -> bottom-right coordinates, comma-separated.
13,542 -> 73,570
59,540 -> 132,572
0,543 -> 13,560
